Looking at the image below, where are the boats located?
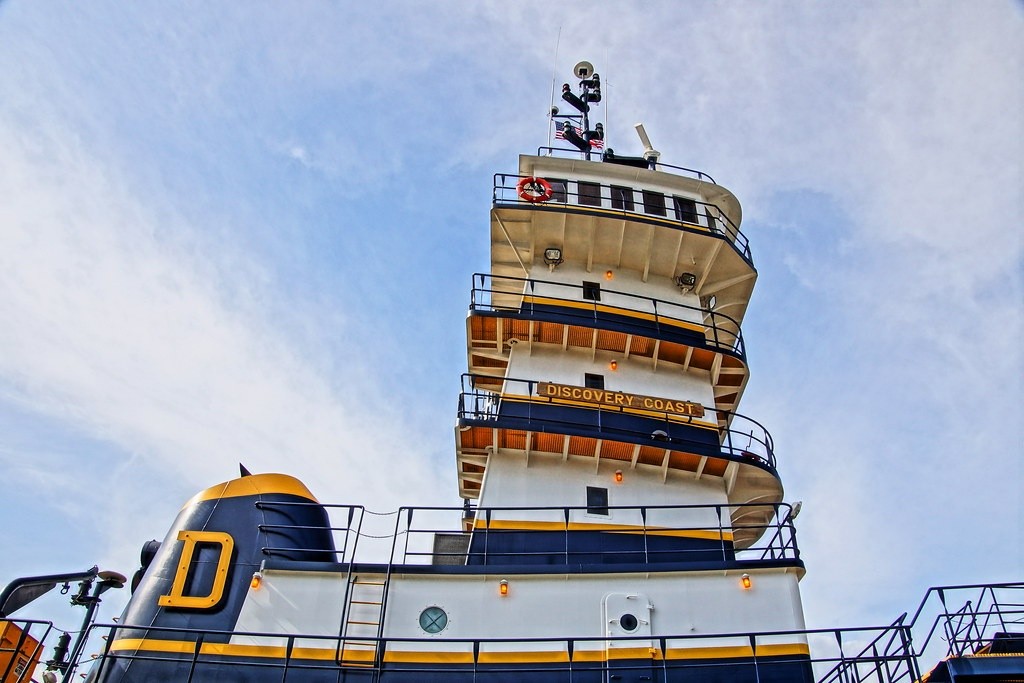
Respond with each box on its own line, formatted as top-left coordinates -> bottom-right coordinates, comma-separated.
0,25 -> 1024,683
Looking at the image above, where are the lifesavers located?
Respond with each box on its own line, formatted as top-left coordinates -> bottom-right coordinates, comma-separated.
516,177 -> 552,201
